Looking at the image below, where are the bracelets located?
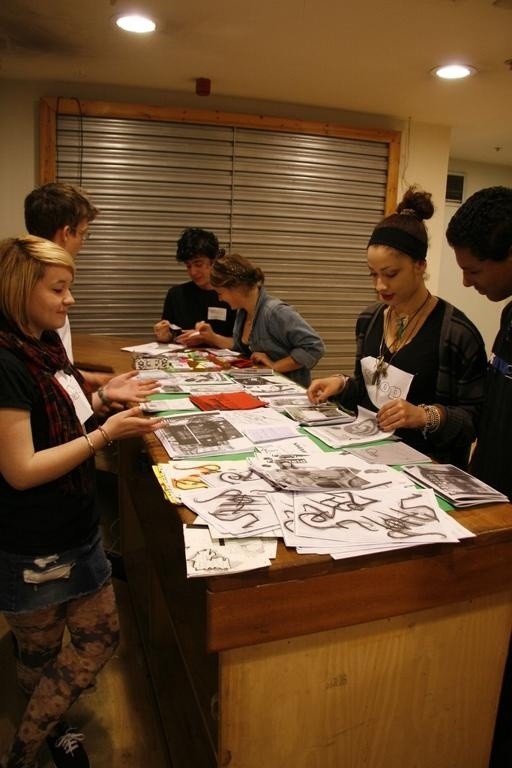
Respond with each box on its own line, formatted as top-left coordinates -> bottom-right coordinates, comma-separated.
84,425 -> 112,454
329,372 -> 349,395
414,399 -> 441,439
97,387 -> 112,406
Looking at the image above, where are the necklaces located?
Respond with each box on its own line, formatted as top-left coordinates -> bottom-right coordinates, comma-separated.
246,313 -> 254,327
369,289 -> 433,386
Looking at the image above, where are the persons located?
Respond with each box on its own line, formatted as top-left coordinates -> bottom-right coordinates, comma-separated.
0,231 -> 164,768
24,183 -> 116,387
445,185 -> 512,505
153,226 -> 234,353
309,191 -> 487,470
194,252 -> 325,393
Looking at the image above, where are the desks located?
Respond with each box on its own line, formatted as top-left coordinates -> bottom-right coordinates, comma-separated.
72,332 -> 511,767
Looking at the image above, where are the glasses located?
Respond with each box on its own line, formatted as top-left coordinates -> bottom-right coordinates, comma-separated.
73,226 -> 91,240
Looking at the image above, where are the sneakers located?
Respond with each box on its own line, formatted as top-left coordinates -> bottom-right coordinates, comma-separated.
46,720 -> 90,768
